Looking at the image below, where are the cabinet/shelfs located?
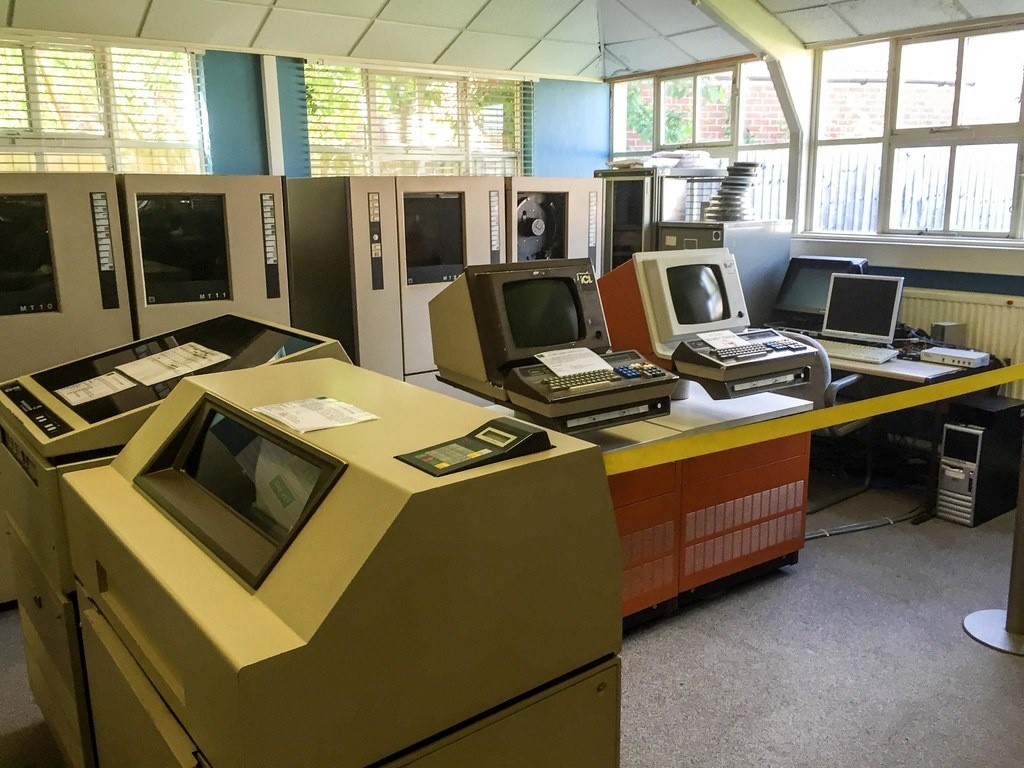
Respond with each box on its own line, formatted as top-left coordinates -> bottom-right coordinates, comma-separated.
607,429 -> 811,617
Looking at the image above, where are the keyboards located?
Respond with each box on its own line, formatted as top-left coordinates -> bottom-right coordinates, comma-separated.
814,340 -> 899,363
505,349 -> 679,418
672,327 -> 819,381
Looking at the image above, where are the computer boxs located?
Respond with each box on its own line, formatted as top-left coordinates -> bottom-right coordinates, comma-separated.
935,420 -> 1024,528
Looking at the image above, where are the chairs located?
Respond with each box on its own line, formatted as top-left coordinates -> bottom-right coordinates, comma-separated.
778,330 -> 881,516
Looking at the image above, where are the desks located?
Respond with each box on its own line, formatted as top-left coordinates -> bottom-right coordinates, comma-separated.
827,351 -> 1010,526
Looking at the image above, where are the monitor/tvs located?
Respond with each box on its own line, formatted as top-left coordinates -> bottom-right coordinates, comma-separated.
428,257 -> 611,405
598,247 -> 751,371
821,273 -> 905,344
774,255 -> 869,330
